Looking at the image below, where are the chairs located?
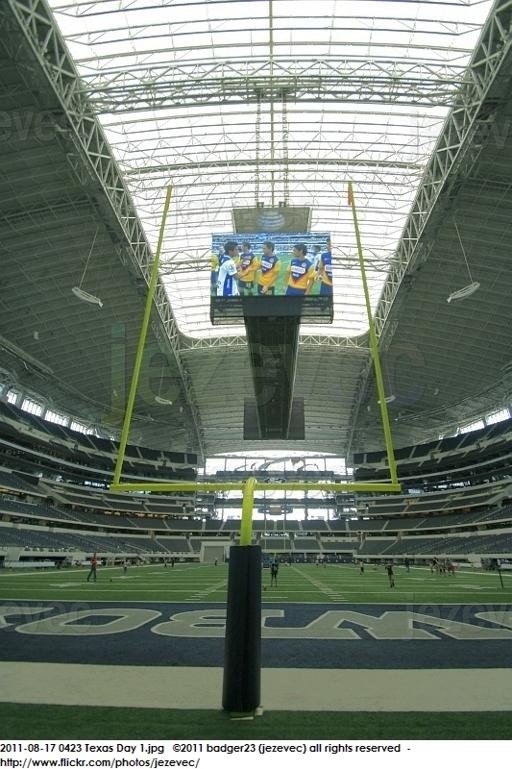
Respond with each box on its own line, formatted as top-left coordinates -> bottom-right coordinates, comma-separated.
0,469 -> 512,555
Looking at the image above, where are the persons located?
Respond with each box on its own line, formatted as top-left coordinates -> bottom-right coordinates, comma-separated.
402,558 -> 409,573
215,557 -> 217,566
87,553 -> 97,582
384,560 -> 395,588
315,559 -> 318,568
311,245 -> 323,282
171,558 -> 174,568
360,560 -> 365,576
123,560 -> 129,575
318,238 -> 332,296
270,559 -> 278,587
164,558 -> 167,569
258,241 -> 281,296
210,242 -> 257,297
430,557 -> 456,578
282,244 -> 317,296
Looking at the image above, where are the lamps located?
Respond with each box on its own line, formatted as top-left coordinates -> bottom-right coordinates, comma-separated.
152,361 -> 173,405
446,220 -> 480,304
378,360 -> 396,405
70,225 -> 105,308
